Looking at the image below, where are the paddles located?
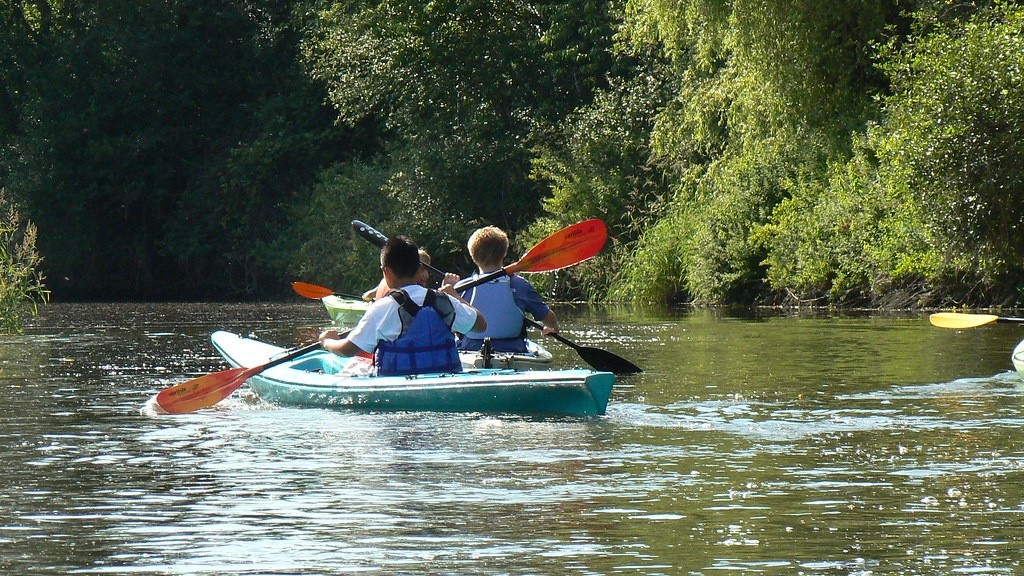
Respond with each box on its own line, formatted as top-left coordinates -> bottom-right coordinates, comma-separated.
351,217 -> 643,377
929,312 -> 1024,330
291,280 -> 375,302
157,219 -> 608,416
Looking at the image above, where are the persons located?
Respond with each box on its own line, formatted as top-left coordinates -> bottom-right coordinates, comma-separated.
442,227 -> 559,354
319,236 -> 487,373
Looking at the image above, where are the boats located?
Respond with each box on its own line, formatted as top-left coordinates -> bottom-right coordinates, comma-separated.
210,329 -> 617,418
1011,337 -> 1024,377
321,294 -> 366,325
